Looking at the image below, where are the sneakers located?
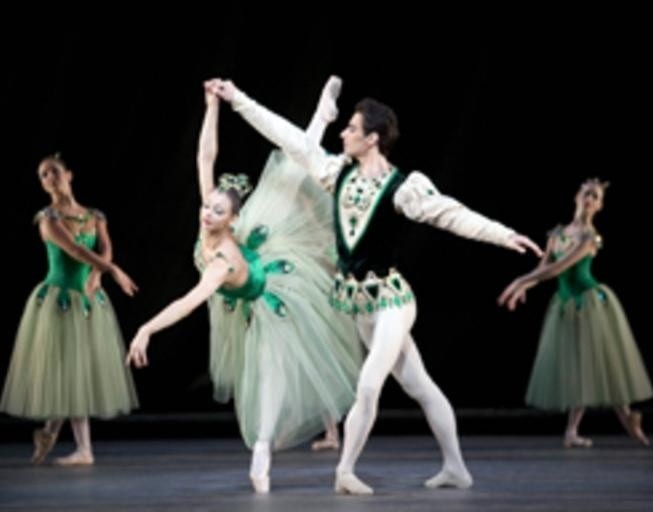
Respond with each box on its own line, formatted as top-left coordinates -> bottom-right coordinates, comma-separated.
318,77 -> 342,121
426,470 -> 472,490
334,472 -> 374,496
627,413 -> 650,446
29,430 -> 93,464
313,439 -> 341,450
565,435 -> 591,449
249,444 -> 270,493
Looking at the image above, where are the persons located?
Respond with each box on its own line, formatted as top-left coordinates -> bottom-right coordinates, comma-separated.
204,76 -> 545,497
269,183 -> 343,452
125,71 -> 363,490
495,172 -> 651,448
0,153 -> 145,469
